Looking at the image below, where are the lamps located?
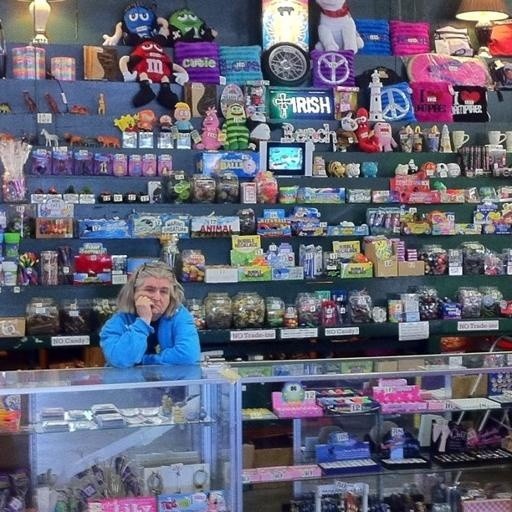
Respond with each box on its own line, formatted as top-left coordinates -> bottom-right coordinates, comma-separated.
28,1 -> 51,45
453,2 -> 512,60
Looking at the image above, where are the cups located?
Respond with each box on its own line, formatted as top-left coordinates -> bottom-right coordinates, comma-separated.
487,130 -> 512,152
449,130 -> 469,153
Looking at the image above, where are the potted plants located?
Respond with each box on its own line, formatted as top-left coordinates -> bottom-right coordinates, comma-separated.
29,185 -> 97,206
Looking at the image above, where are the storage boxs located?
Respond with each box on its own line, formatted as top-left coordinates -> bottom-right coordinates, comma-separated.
365,239 -> 425,276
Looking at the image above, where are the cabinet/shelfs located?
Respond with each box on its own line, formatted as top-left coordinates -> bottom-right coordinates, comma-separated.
1,44 -> 512,349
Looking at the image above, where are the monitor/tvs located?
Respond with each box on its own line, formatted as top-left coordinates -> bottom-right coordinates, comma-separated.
260,141 -> 313,178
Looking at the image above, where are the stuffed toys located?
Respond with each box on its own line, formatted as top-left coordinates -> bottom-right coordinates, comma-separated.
334,112 -> 397,153
0,0 -> 512,179
316,0 -> 364,54
102,0 -> 270,150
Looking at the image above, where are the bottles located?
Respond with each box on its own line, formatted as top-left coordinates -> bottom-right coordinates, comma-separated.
282,303 -> 299,328
94,297 -> 120,336
231,290 -> 265,329
183,298 -> 206,331
24,295 -> 62,336
189,173 -> 217,203
61,297 -> 94,335
203,292 -> 233,330
294,292 -> 323,328
216,169 -> 241,204
163,170 -> 192,204
264,296 -> 285,328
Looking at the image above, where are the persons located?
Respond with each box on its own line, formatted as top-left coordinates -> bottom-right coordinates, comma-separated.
98,259 -> 203,368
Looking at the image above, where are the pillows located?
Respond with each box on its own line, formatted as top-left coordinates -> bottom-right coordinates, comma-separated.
354,19 -> 432,57
172,41 -> 264,88
379,83 -> 490,126
309,49 -> 358,90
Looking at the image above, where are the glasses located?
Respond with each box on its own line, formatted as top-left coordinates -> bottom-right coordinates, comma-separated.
142,261 -> 173,272
